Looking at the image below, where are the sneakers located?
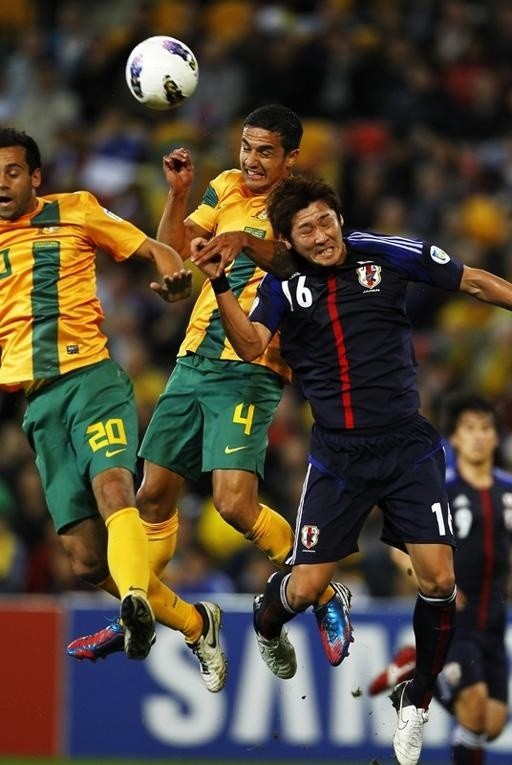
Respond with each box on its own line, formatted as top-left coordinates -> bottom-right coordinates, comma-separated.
65,616 -> 126,664
389,677 -> 430,765
118,591 -> 158,662
310,580 -> 355,669
368,645 -> 416,698
184,599 -> 228,694
251,592 -> 299,680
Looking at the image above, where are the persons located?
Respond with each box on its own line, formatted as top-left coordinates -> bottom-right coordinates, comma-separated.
0,1 -> 511,765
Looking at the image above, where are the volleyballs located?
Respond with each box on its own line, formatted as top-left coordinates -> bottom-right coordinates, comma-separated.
125,37 -> 199,106
